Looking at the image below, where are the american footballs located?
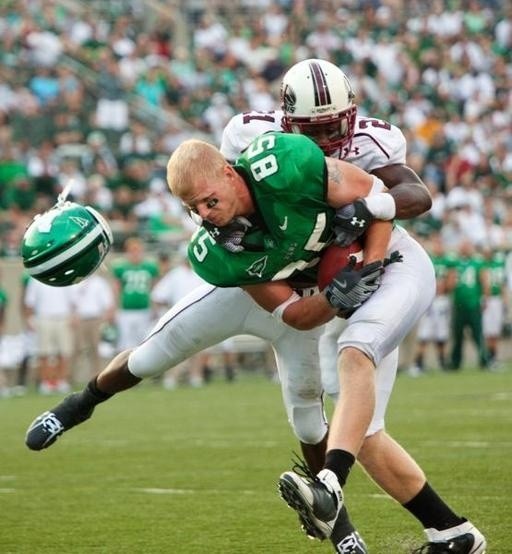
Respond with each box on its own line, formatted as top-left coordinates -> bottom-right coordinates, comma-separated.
316,237 -> 364,294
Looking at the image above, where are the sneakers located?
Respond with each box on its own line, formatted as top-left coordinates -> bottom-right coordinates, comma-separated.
278,450 -> 345,543
23,390 -> 95,451
329,505 -> 368,554
412,516 -> 487,554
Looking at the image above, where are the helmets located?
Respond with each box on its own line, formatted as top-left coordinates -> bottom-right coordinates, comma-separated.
279,58 -> 358,161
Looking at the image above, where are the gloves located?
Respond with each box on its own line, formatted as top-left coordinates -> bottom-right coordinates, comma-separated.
328,191 -> 374,248
323,255 -> 384,320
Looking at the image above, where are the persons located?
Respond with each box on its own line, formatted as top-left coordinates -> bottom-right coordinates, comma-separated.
0,0 -> 512,395
167,129 -> 490,554
24,58 -> 433,554
